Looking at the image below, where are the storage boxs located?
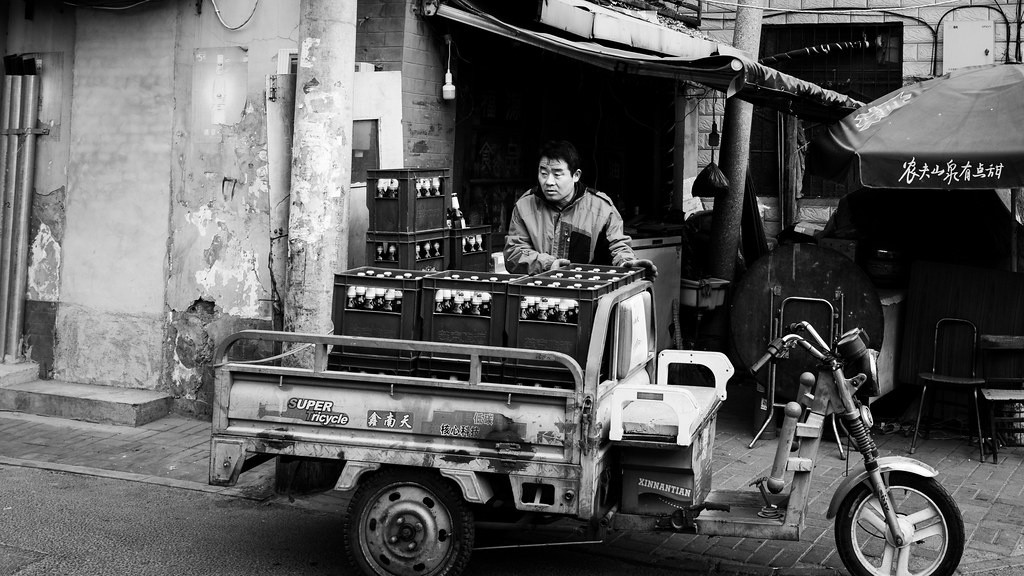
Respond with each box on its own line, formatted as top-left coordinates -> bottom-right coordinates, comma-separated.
329,263 -> 646,389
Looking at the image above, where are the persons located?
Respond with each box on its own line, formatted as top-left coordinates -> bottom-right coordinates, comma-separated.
503,139 -> 659,283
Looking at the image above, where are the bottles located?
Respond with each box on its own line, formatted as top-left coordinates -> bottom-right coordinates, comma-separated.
347,178 -> 636,323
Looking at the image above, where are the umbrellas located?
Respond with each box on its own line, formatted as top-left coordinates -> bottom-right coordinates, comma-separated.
828,64 -> 1022,278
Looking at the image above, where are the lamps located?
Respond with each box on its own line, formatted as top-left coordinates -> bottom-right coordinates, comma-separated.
444,70 -> 457,99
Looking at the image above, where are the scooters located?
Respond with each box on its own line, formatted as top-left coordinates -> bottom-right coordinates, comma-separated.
208,275 -> 967,576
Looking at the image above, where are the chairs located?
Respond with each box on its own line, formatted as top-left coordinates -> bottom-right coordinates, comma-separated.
908,317 -> 989,463
611,346 -> 736,515
979,332 -> 1024,464
